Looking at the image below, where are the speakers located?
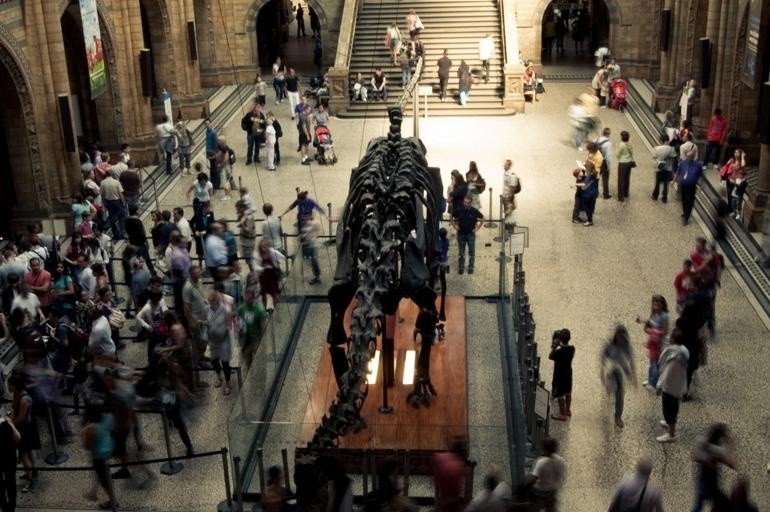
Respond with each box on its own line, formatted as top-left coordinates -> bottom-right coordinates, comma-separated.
660,9 -> 672,52
140,48 -> 152,98
759,82 -> 770,146
699,38 -> 711,89
187,21 -> 198,61
57,93 -> 77,153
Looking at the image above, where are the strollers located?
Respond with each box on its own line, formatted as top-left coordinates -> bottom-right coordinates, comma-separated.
313,124 -> 337,166
38,317 -> 90,369
607,78 -> 628,114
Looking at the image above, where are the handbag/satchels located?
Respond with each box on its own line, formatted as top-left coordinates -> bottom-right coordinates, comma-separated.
515,177 -> 521,194
720,164 -> 731,179
477,175 -> 485,192
657,160 -> 667,169
630,160 -> 637,169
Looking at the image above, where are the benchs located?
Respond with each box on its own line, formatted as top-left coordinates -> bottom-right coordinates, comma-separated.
319,95 -> 330,105
525,91 -> 535,104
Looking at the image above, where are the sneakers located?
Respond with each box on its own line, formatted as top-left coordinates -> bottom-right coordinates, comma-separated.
643,381 -> 660,393
574,217 -> 594,226
656,432 -> 676,442
99,502 -> 118,509
552,408 -> 571,421
660,419 -> 671,427
216,377 -> 231,396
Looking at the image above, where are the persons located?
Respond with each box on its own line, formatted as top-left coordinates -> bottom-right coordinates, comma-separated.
307,5 -> 321,40
350,72 -> 372,103
384,3 -> 731,170
368,68 -> 389,103
319,194 -> 769,512
436,108 -> 750,234
296,3 -> 309,40
1,56 -> 338,511
